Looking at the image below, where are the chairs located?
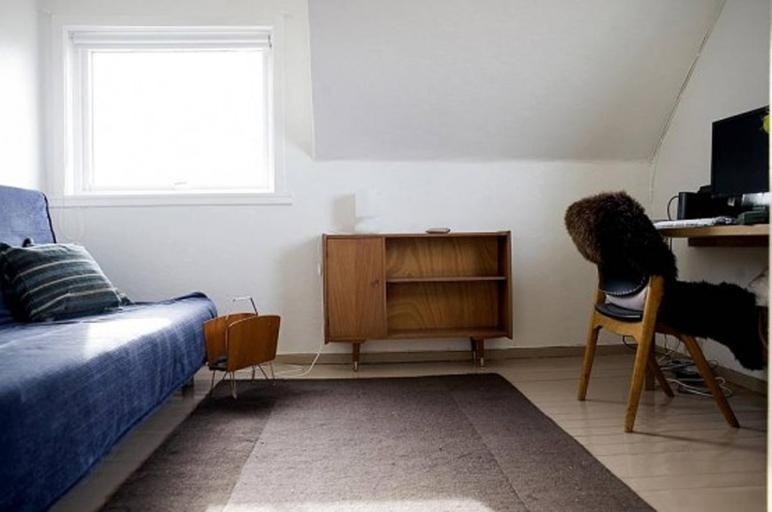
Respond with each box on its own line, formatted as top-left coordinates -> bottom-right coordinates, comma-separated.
564,194 -> 767,434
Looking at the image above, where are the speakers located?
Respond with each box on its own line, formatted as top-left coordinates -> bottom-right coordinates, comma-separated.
678,192 -> 697,220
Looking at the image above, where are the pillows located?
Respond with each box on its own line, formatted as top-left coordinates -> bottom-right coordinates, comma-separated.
3,242 -> 120,321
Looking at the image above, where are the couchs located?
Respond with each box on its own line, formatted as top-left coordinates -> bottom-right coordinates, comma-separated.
0,183 -> 217,512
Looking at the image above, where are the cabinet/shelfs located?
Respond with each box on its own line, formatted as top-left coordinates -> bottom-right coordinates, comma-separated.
321,230 -> 514,371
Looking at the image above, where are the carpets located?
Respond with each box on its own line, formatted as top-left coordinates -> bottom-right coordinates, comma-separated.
94,371 -> 658,512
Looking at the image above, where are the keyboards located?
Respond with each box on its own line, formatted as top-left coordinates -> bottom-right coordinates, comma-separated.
653,218 -> 717,229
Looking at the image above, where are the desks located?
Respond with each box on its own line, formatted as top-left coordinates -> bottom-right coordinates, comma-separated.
646,214 -> 770,398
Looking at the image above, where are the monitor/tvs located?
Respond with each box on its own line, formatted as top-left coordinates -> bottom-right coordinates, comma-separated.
711,104 -> 770,219
